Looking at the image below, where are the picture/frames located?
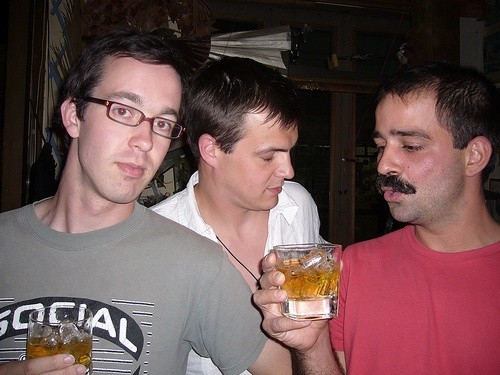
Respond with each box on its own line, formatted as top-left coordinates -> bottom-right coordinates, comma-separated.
20,0 -> 82,208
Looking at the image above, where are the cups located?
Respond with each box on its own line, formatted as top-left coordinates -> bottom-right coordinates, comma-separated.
274,244 -> 338,319
26,305 -> 93,375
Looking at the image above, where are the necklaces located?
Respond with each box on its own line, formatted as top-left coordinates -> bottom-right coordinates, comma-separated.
216,235 -> 259,284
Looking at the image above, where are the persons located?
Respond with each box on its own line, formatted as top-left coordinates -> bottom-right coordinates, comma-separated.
0,31 -> 297,375
145,57 -> 331,374
253,62 -> 500,375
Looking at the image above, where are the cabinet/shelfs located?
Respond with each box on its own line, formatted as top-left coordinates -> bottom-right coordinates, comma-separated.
460,18 -> 500,221
211,0 -> 433,247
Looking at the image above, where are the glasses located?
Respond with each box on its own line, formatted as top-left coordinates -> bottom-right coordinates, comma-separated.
76,94 -> 188,142
